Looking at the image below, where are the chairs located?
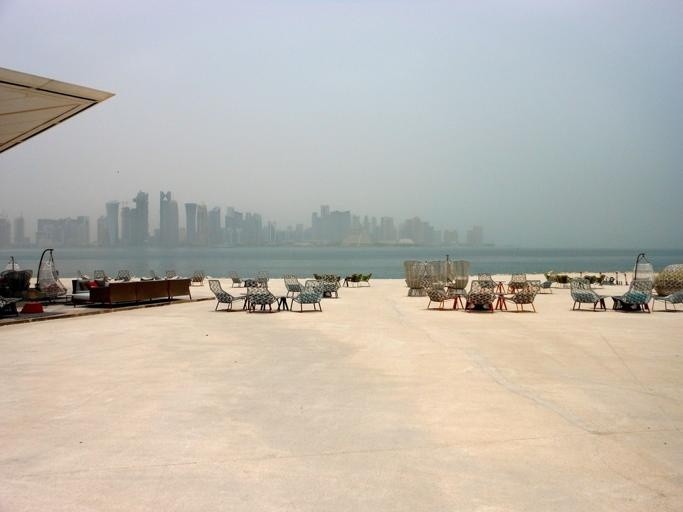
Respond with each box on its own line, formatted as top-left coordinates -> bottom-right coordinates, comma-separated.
93,266 -> 373,314
404,251 -> 683,315
